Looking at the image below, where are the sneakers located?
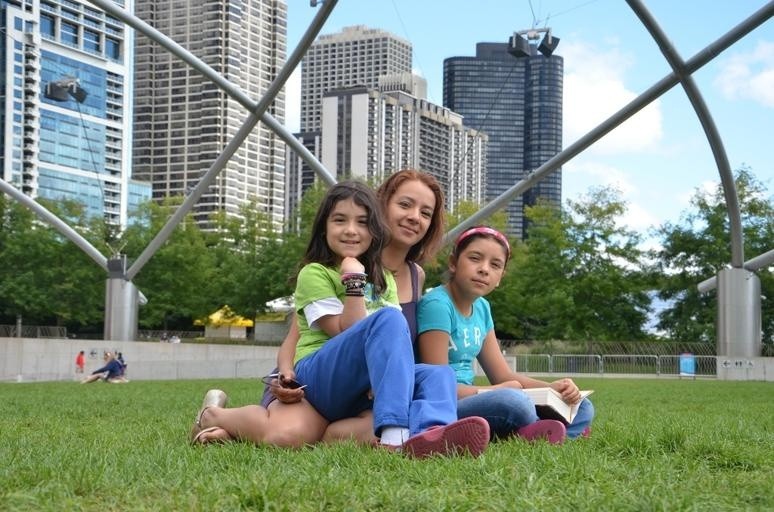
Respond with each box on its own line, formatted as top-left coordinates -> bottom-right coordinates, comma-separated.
517,419 -> 566,444
372,416 -> 490,461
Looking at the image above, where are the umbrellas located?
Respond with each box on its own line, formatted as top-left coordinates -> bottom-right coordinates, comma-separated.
192,305 -> 253,338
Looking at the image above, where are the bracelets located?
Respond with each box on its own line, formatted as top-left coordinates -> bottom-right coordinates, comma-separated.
341,272 -> 368,297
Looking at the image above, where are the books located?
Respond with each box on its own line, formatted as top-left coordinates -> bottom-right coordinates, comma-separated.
474,384 -> 594,426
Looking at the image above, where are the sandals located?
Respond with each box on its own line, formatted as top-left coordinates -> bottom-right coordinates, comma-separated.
189,388 -> 228,446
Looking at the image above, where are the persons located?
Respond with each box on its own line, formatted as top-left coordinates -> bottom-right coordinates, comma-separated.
186,170 -> 443,452
75,349 -> 126,386
417,225 -> 595,445
291,181 -> 490,463
